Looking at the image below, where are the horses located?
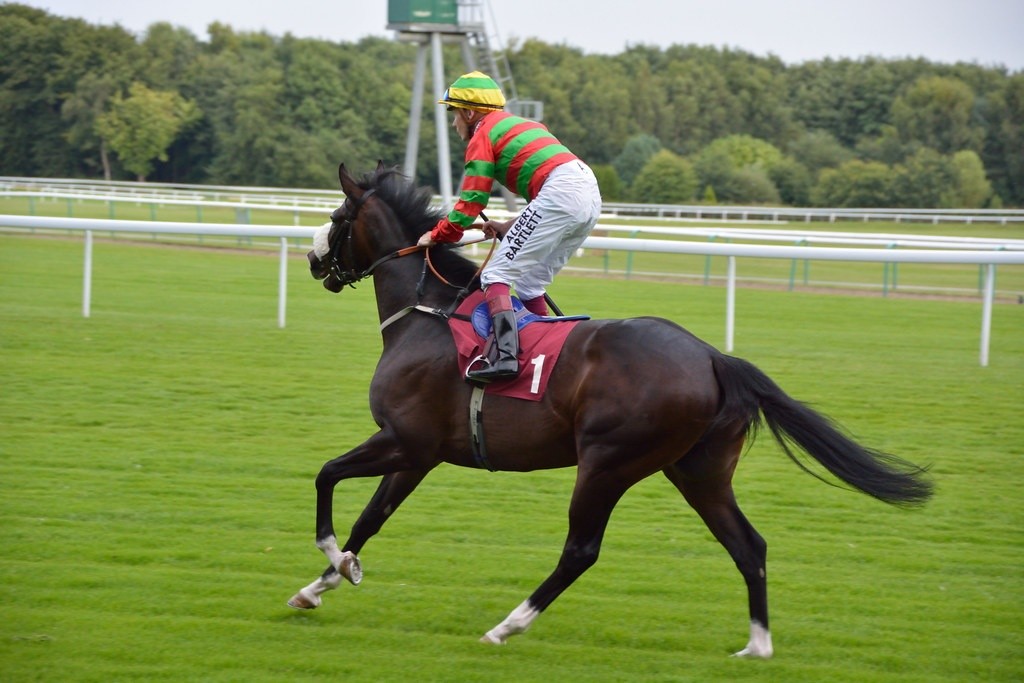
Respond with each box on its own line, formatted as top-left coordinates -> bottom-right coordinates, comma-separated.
289,159 -> 939,660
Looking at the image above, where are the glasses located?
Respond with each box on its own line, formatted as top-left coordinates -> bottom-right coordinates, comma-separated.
443,87 -> 449,101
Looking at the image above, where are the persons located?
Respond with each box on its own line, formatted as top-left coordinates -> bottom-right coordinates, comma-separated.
417,70 -> 602,381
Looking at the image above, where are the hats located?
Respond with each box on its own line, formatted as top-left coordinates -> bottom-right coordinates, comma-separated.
438,70 -> 506,112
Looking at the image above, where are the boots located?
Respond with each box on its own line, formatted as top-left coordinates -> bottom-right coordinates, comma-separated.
464,283 -> 519,384
522,295 -> 549,316
